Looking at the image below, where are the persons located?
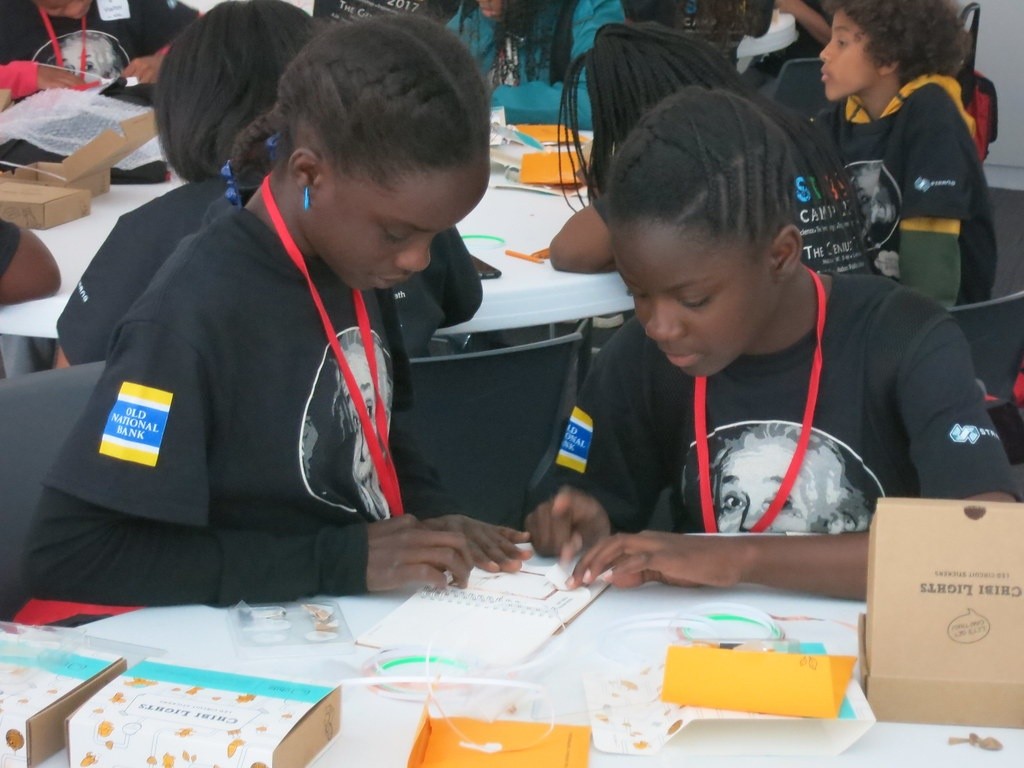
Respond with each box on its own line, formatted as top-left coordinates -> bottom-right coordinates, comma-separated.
813,3 -> 998,309
21,15 -> 531,608
0,0 -> 867,378
524,21 -> 1023,602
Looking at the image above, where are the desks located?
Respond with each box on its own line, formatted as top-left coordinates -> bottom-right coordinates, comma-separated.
0,125 -> 634,340
48,540 -> 1024,768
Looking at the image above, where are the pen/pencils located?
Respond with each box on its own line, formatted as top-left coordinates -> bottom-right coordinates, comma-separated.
505,250 -> 544,264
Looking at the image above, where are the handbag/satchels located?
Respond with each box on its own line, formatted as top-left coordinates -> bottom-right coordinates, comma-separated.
948,3 -> 998,163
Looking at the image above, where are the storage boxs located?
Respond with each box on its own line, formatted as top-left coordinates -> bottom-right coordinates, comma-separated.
855,497 -> 1024,728
65,661 -> 340,768
0,183 -> 91,228
0,111 -> 156,203
0,630 -> 127,768
520,151 -> 582,185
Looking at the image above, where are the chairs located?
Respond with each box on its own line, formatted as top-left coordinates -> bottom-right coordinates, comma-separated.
955,292 -> 1024,458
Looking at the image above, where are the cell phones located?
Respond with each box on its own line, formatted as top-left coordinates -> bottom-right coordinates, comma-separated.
470,253 -> 501,280
